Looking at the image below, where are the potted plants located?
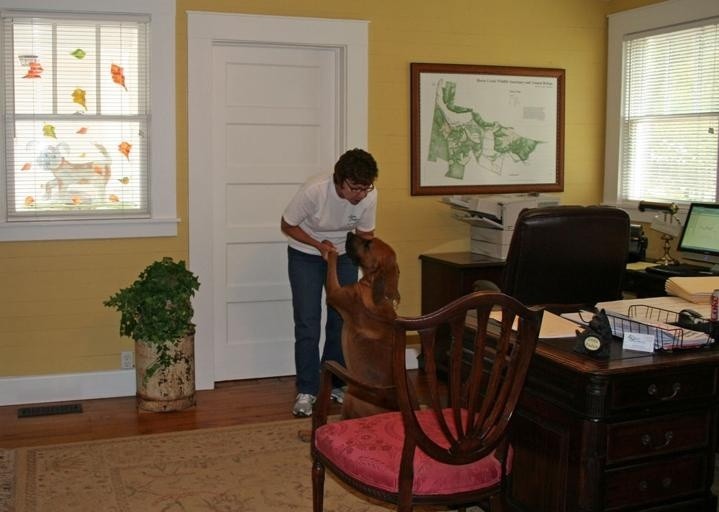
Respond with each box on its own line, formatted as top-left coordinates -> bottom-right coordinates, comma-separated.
103,254 -> 204,413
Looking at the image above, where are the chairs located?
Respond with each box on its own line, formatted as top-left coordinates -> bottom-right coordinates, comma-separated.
305,291 -> 546,510
500,205 -> 631,305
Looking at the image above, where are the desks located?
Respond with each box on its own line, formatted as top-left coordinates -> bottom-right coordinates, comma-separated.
465,295 -> 718,512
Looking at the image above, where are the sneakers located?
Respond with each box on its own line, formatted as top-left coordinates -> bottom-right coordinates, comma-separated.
291,394 -> 317,417
329,388 -> 348,406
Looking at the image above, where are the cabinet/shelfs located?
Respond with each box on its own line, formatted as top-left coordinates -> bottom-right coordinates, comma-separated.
418,251 -> 502,372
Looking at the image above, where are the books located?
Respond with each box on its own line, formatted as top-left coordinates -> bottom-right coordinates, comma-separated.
666,274 -> 719,303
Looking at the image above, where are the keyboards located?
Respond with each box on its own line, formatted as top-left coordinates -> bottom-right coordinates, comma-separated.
645,262 -> 718,277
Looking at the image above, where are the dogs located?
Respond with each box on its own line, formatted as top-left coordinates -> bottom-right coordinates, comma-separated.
297,232 -> 400,442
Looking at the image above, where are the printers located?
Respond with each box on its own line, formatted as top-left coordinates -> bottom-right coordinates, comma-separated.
437,194 -> 560,259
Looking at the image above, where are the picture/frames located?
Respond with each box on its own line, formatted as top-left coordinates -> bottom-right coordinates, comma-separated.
408,61 -> 566,196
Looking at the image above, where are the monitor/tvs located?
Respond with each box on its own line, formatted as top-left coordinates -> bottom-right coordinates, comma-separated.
677,203 -> 719,271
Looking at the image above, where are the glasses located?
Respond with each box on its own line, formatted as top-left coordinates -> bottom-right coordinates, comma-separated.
343,178 -> 375,193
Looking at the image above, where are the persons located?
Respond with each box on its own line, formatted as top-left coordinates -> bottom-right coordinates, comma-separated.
279,147 -> 378,418
